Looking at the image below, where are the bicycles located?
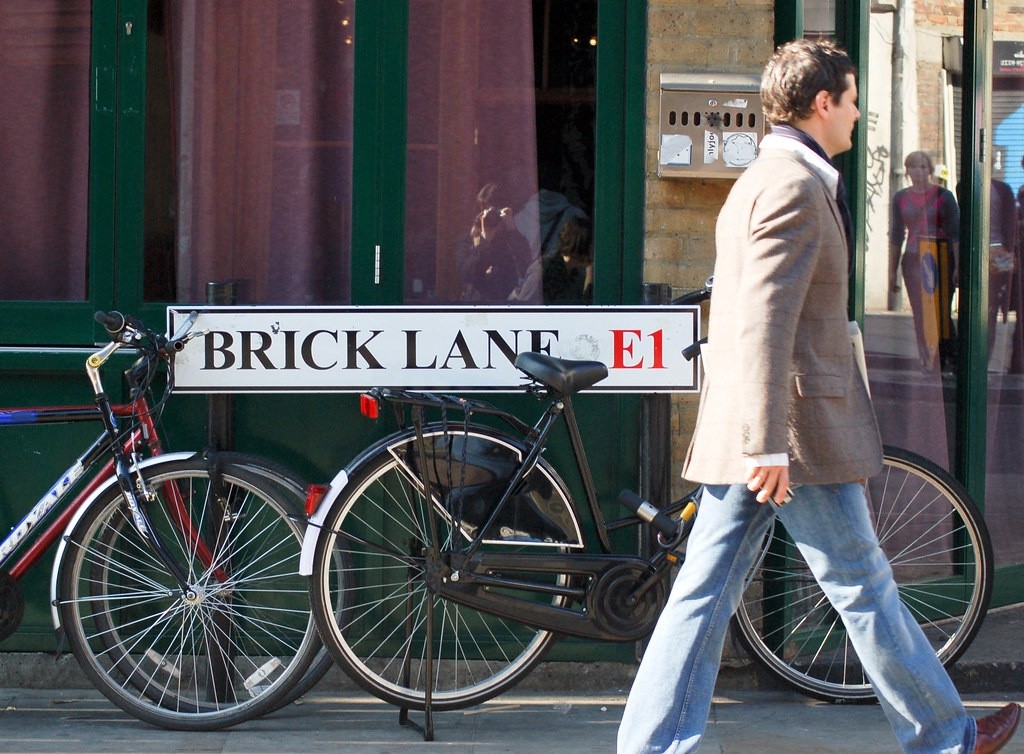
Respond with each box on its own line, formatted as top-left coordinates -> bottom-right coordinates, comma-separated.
0,304 -> 363,732
284,264 -> 998,744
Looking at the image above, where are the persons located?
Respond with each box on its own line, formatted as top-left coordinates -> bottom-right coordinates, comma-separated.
889,151 -> 1024,374
616,37 -> 1021,754
458,151 -> 593,302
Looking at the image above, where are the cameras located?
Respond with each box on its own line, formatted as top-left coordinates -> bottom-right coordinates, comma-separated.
486,210 -> 502,227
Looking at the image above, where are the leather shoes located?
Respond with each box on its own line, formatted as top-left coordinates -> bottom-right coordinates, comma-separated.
974,703 -> 1021,754
